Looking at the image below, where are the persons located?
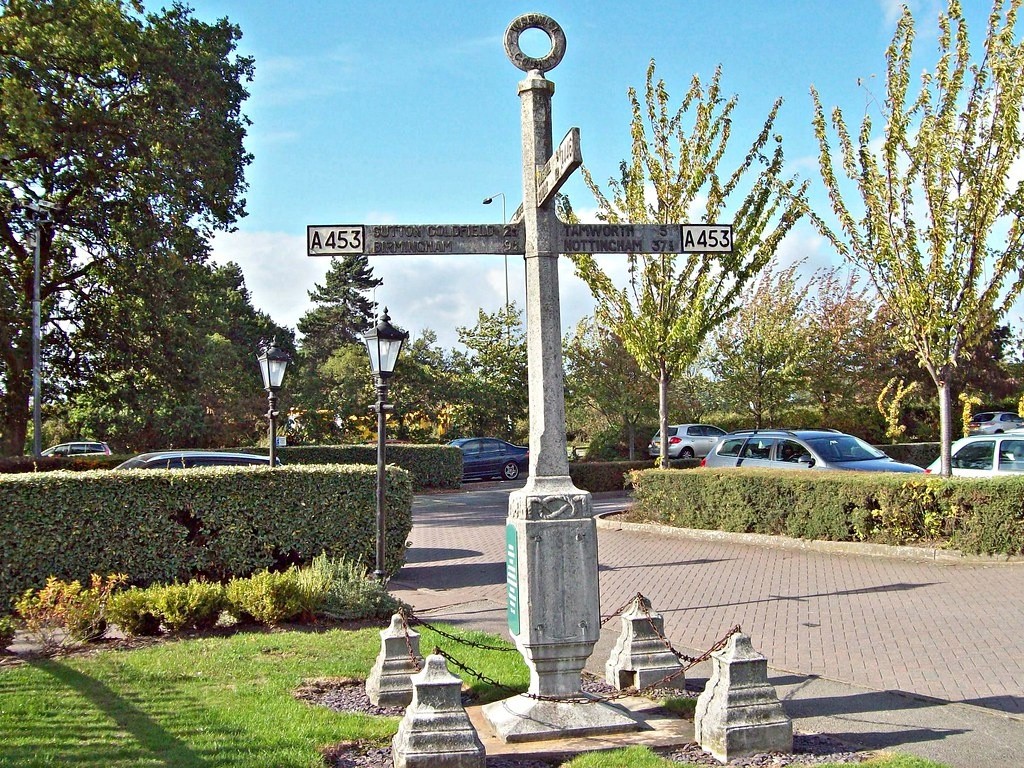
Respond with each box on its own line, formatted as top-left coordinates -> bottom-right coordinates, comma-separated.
781,445 -> 798,463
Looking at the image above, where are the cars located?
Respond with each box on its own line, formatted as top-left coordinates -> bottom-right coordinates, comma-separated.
40,441 -> 113,457
967,410 -> 1024,435
108,451 -> 284,467
919,437 -> 1024,479
445,437 -> 530,480
648,423 -> 728,460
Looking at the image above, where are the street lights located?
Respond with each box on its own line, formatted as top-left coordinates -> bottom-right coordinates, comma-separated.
482,191 -> 513,355
360,305 -> 410,584
254,335 -> 290,467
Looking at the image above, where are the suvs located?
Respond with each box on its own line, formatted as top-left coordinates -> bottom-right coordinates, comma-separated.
700,425 -> 925,475
